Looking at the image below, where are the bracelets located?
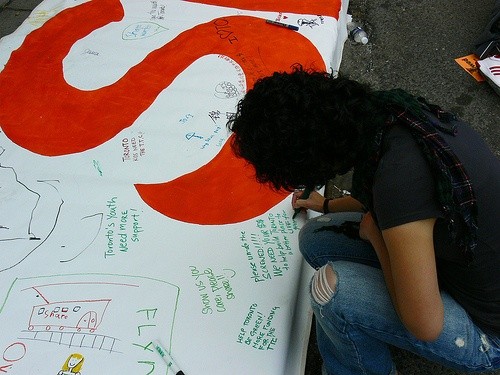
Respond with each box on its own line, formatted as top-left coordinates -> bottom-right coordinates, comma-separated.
323,197 -> 334,214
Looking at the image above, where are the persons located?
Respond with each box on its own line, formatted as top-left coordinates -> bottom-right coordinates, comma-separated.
231,61 -> 500,375
472,9 -> 500,97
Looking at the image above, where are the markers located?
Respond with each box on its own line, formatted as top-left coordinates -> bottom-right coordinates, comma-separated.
292,184 -> 314,220
